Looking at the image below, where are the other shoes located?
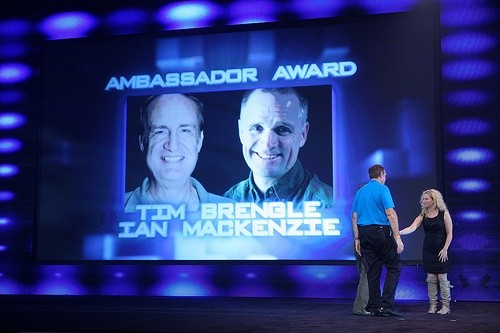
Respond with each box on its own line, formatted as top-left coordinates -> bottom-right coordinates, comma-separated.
355,307 -> 371,315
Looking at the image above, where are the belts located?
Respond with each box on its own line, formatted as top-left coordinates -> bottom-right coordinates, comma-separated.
358,227 -> 389,230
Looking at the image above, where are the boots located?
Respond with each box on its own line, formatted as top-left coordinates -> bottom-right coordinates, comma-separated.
436,281 -> 454,315
427,279 -> 437,314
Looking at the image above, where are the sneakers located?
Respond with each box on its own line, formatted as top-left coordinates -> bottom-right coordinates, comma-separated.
367,307 -> 379,316
379,307 -> 407,318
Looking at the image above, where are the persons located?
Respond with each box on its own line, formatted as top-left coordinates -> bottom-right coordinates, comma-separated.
124,93 -> 241,212
352,245 -> 371,316
222,87 -> 333,209
352,164 -> 405,320
399,189 -> 453,315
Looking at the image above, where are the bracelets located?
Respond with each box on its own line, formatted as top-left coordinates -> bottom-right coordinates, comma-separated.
395,235 -> 400,239
354,236 -> 360,240
